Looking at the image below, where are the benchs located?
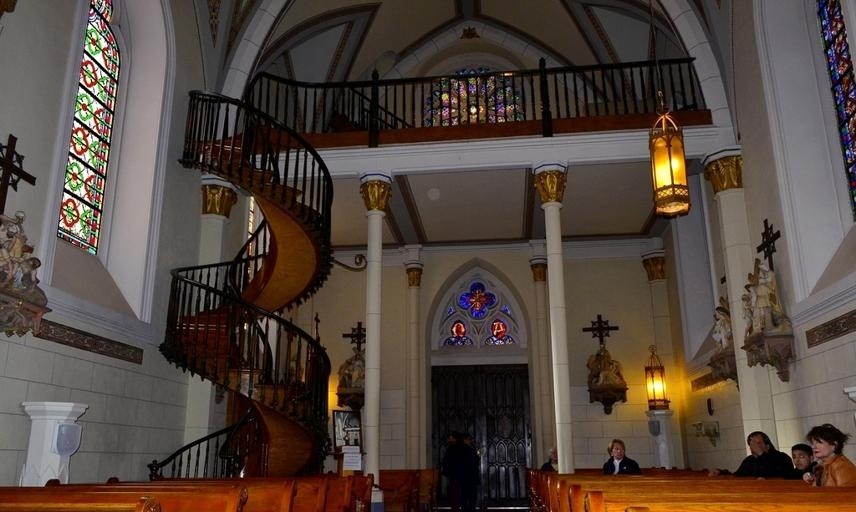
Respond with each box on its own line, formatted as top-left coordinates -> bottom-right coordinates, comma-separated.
0,469 -> 438,511
524,465 -> 855,512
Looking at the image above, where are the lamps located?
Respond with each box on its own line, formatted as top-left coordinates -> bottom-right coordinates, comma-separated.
648,0 -> 692,218
643,281 -> 671,412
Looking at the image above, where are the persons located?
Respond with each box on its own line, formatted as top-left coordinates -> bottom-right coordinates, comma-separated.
603,439 -> 642,475
542,446 -> 558,471
443,430 -> 479,512
802,422 -> 855,487
0,208 -> 41,290
464,431 -> 473,445
740,262 -> 786,336
708,431 -> 795,479
786,442 -> 818,478
